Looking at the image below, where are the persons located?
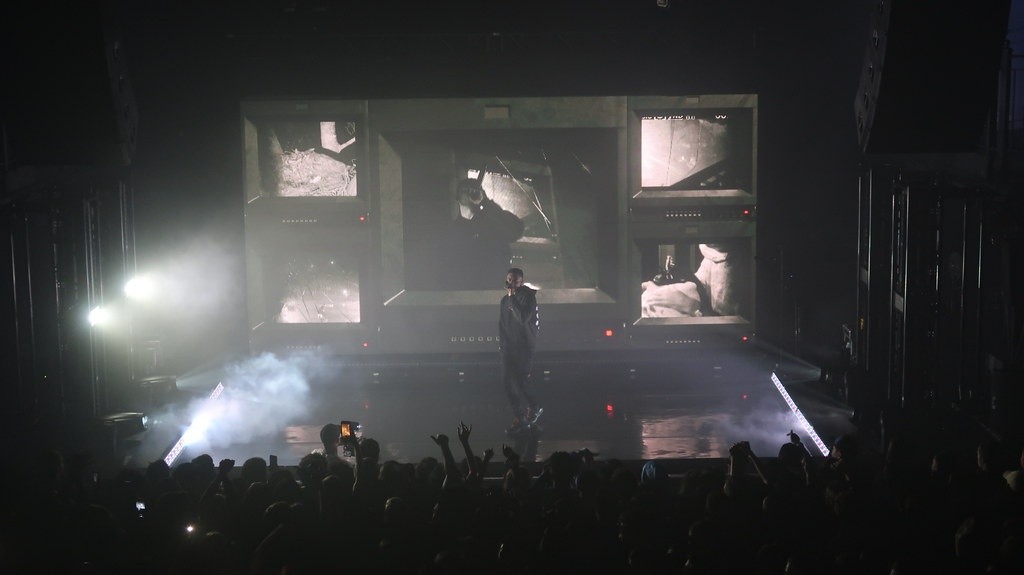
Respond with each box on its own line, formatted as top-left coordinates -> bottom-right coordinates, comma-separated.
0,420 -> 1023,575
499,268 -> 543,432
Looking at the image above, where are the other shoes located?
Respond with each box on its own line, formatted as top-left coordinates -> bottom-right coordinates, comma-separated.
505,415 -> 523,436
522,404 -> 544,431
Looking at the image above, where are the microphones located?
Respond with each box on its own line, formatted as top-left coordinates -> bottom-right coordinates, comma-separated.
504,280 -> 512,288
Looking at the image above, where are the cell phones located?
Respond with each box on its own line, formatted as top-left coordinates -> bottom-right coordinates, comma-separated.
270,456 -> 277,467
341,421 -> 351,437
136,500 -> 146,510
